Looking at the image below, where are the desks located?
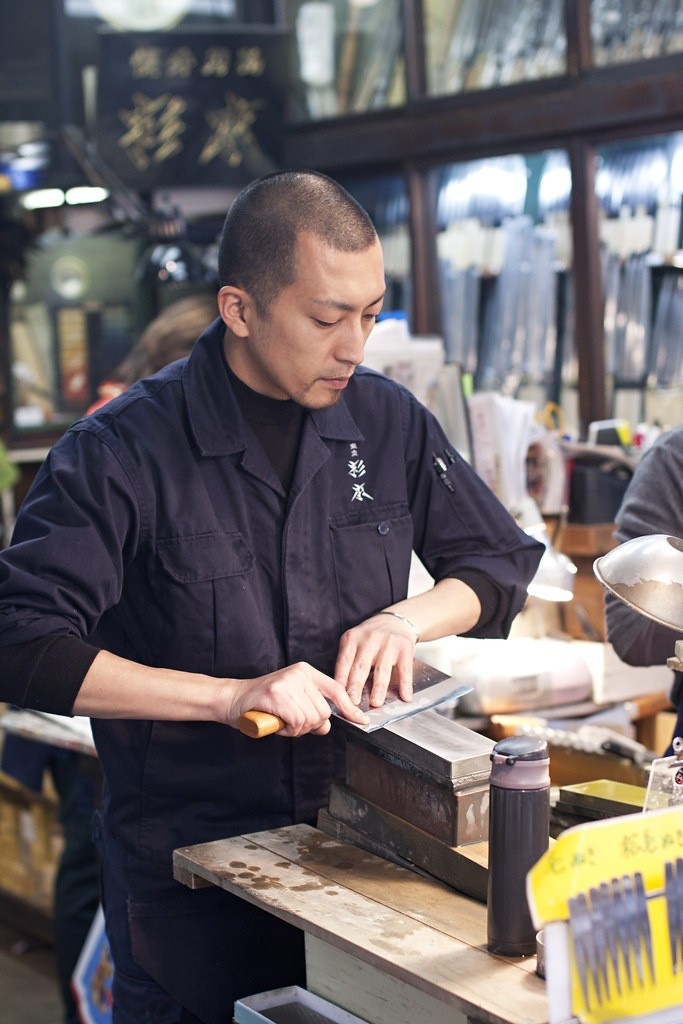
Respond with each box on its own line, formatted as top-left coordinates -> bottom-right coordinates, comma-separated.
172,782 -> 561,1024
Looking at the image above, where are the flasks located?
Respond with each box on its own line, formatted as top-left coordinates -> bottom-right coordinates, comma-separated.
487,735 -> 551,957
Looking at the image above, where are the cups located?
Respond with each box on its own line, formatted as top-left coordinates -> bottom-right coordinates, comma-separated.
535,929 -> 547,979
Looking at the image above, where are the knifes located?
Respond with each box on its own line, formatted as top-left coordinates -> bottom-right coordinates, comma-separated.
601,740 -> 661,763
235,658 -> 476,738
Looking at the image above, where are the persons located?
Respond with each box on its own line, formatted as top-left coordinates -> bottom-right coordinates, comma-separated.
605,426 -> 683,757
0,169 -> 547,1023
54,292 -> 220,1023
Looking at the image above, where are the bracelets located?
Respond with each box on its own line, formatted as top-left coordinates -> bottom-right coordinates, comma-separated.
376,612 -> 419,643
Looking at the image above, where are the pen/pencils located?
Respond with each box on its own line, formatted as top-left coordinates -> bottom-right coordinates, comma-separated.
431,447 -> 460,493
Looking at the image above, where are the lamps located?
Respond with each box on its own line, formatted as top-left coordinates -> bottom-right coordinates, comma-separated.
65,170 -> 113,207
20,171 -> 67,212
592,534 -> 683,632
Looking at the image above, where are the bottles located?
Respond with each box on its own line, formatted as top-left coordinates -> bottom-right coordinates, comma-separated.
628,422 -> 652,460
